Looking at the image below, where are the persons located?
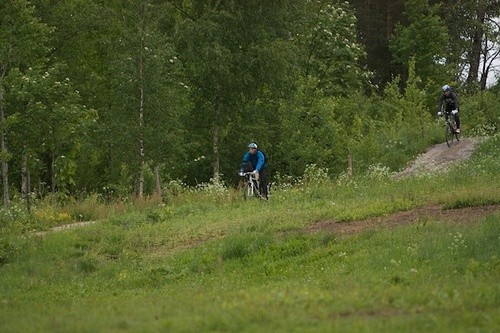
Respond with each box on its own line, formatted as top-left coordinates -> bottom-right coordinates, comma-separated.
240,143 -> 268,199
438,85 -> 461,133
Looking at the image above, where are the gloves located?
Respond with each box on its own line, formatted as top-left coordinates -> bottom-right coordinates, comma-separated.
437,112 -> 442,116
452,110 -> 458,115
253,170 -> 259,174
239,172 -> 244,176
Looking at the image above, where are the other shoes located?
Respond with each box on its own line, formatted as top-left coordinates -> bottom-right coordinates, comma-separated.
455,129 -> 460,133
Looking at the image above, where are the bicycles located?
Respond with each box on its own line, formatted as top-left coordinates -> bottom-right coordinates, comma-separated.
239,170 -> 269,202
437,109 -> 463,148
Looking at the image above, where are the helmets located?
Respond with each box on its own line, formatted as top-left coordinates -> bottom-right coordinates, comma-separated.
248,142 -> 257,149
442,85 -> 450,92
